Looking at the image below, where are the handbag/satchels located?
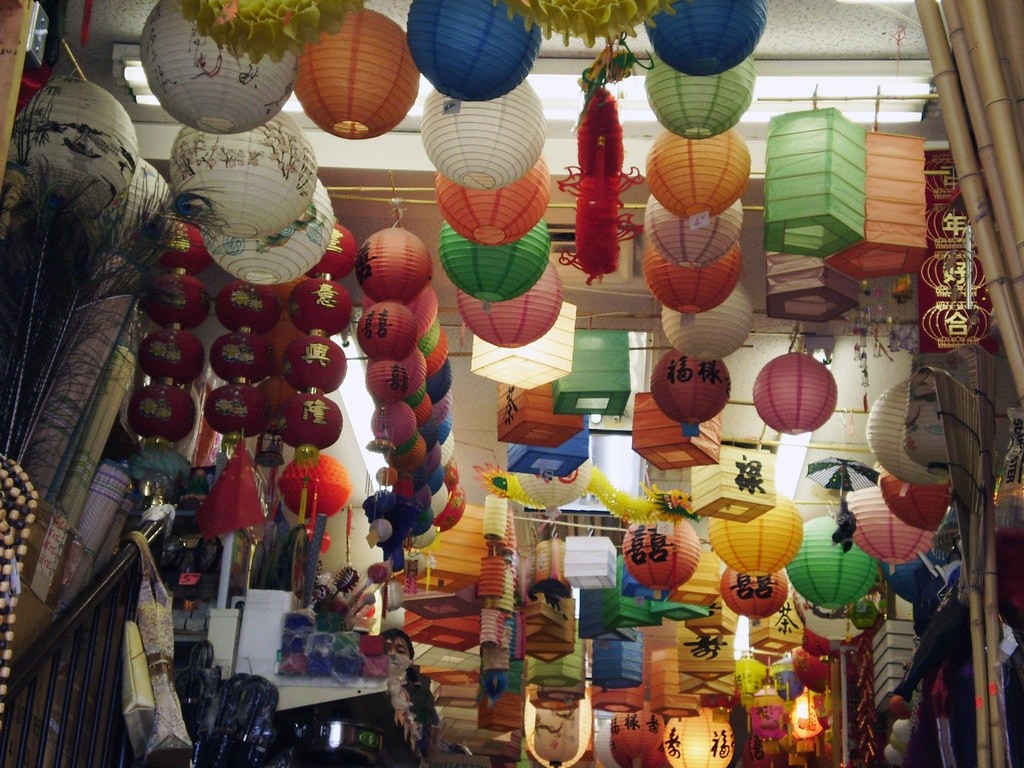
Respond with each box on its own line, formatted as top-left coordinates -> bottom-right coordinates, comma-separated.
120,529 -> 195,768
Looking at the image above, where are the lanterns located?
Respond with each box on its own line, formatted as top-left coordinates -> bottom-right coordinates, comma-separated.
0,0 -> 1024,768
